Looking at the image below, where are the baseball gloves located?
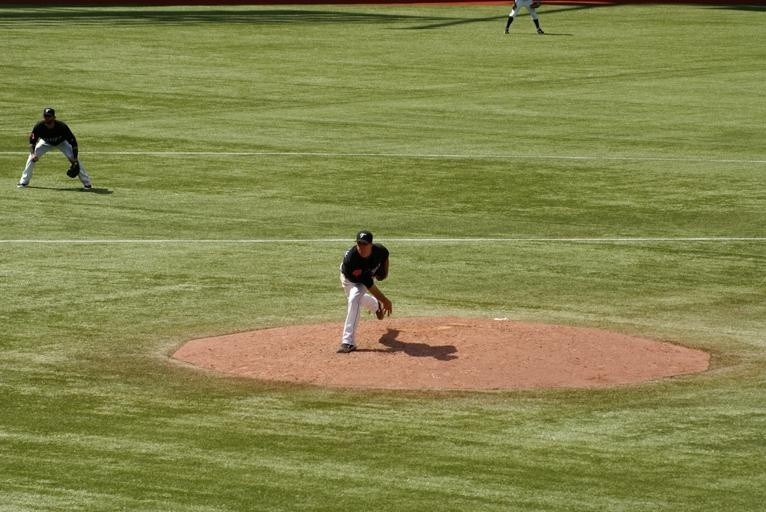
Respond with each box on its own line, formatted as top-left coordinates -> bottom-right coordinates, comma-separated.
376,257 -> 388,280
530,1 -> 541,8
67,161 -> 79,178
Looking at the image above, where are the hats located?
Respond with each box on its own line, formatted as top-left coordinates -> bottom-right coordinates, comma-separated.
354,231 -> 373,245
44,107 -> 55,117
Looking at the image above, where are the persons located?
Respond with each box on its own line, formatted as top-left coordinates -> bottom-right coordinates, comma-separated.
16,108 -> 92,190
504,0 -> 544,34
337,230 -> 393,354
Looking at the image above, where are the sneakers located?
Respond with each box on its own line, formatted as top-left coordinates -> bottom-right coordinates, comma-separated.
375,301 -> 385,321
336,343 -> 357,353
15,184 -> 28,188
84,184 -> 92,190
